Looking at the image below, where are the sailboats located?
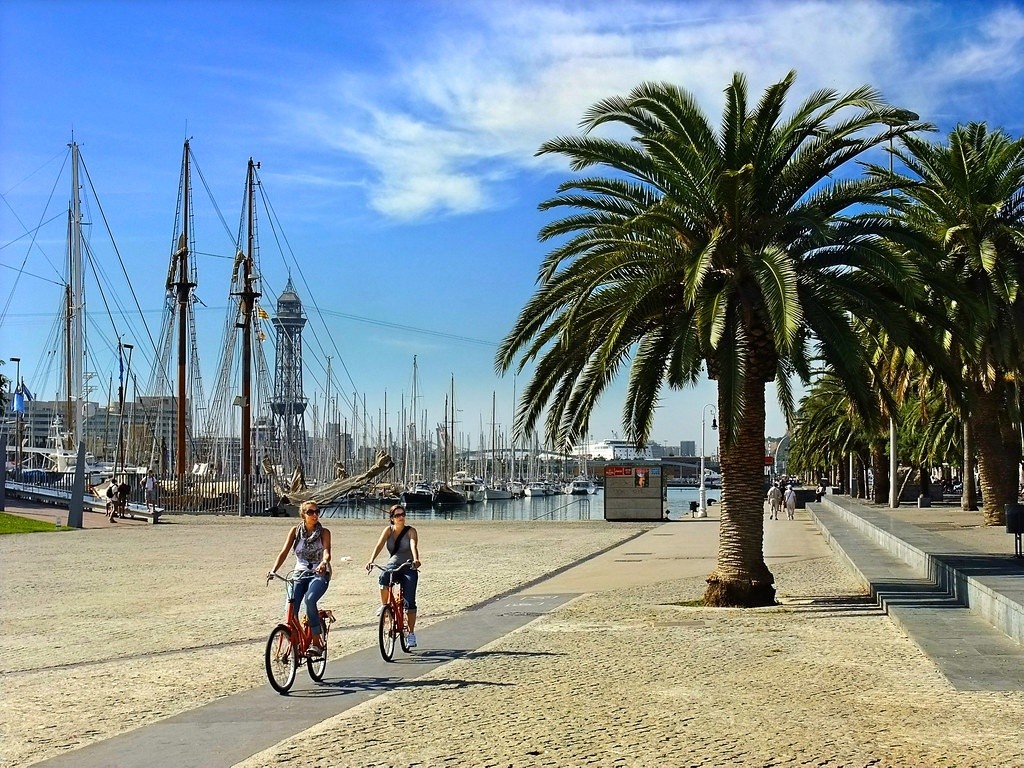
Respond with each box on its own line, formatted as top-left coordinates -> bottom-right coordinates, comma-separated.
568,446 -> 588,495
432,374 -> 467,506
266,569 -> 336,693
399,354 -> 432,508
524,431 -> 546,497
484,391 -> 511,499
336,392 -> 474,502
506,375 -> 524,496
463,415 -> 485,501
0,124 -> 393,515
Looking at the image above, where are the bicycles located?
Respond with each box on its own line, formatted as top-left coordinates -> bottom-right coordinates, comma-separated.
368,559 -> 420,661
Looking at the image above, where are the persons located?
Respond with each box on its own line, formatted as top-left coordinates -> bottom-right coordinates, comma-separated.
816,483 -> 824,503
366,504 -> 422,647
784,485 -> 796,520
776,482 -> 787,512
767,483 -> 782,520
266,500 -> 332,657
105,479 -> 126,523
140,470 -> 159,512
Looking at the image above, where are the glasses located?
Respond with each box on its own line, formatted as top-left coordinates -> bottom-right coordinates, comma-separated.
392,513 -> 405,518
304,509 -> 319,516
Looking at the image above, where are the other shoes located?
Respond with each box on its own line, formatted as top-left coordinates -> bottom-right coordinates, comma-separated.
307,646 -> 322,656
774,518 -> 778,520
770,515 -> 772,519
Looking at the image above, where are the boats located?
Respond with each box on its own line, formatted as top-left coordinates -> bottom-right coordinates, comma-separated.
587,487 -> 598,494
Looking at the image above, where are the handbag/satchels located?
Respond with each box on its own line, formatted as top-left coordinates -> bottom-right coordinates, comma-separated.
784,502 -> 787,508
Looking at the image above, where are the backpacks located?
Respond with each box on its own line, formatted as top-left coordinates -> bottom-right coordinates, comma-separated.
106,484 -> 116,498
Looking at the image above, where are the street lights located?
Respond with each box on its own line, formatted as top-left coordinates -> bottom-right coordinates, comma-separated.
697,404 -> 717,518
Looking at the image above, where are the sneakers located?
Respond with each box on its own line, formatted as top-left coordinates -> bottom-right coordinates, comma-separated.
407,633 -> 416,647
376,604 -> 388,616
